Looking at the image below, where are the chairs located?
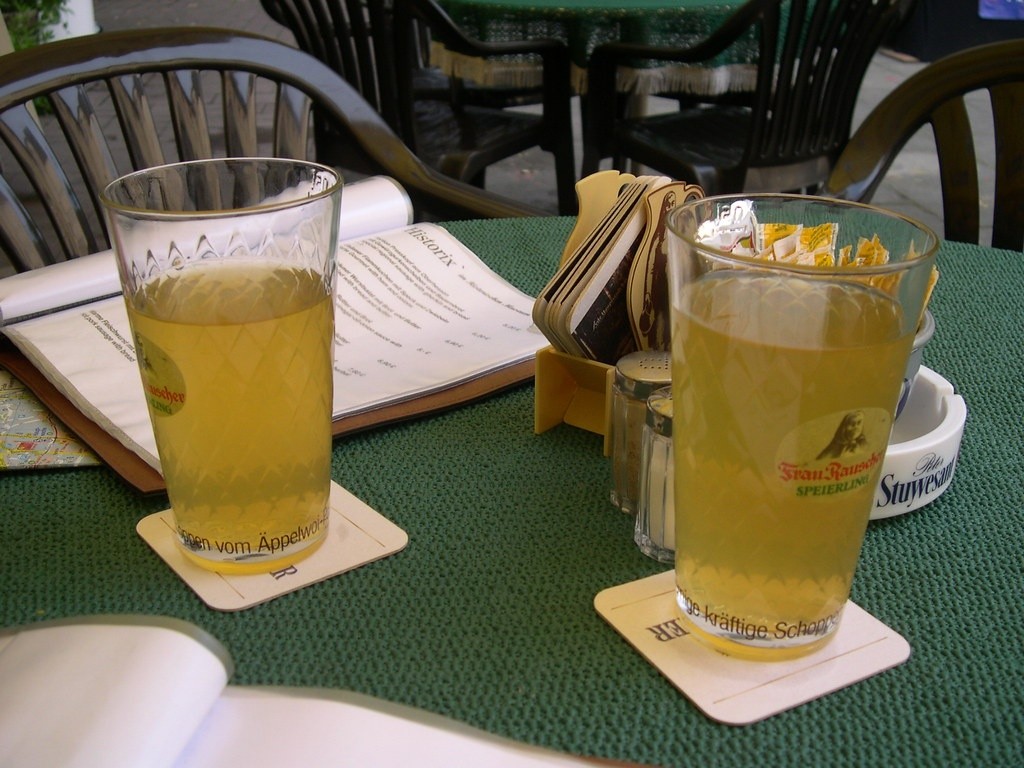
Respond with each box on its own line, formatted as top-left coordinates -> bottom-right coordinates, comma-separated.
585,1 -> 1023,251
0,28 -> 562,284
261,0 -> 578,224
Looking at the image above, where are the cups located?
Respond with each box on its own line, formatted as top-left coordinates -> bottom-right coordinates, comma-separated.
98,156 -> 342,576
666,192 -> 941,658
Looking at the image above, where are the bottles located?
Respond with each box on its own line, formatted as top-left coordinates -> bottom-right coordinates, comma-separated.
633,385 -> 676,565
606,350 -> 672,515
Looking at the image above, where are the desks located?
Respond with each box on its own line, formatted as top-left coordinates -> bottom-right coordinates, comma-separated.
0,219 -> 1023,767
421,0 -> 845,218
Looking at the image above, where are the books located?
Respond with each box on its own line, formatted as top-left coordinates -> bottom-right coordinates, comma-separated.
0,171 -> 556,495
0,615 -> 648,768
